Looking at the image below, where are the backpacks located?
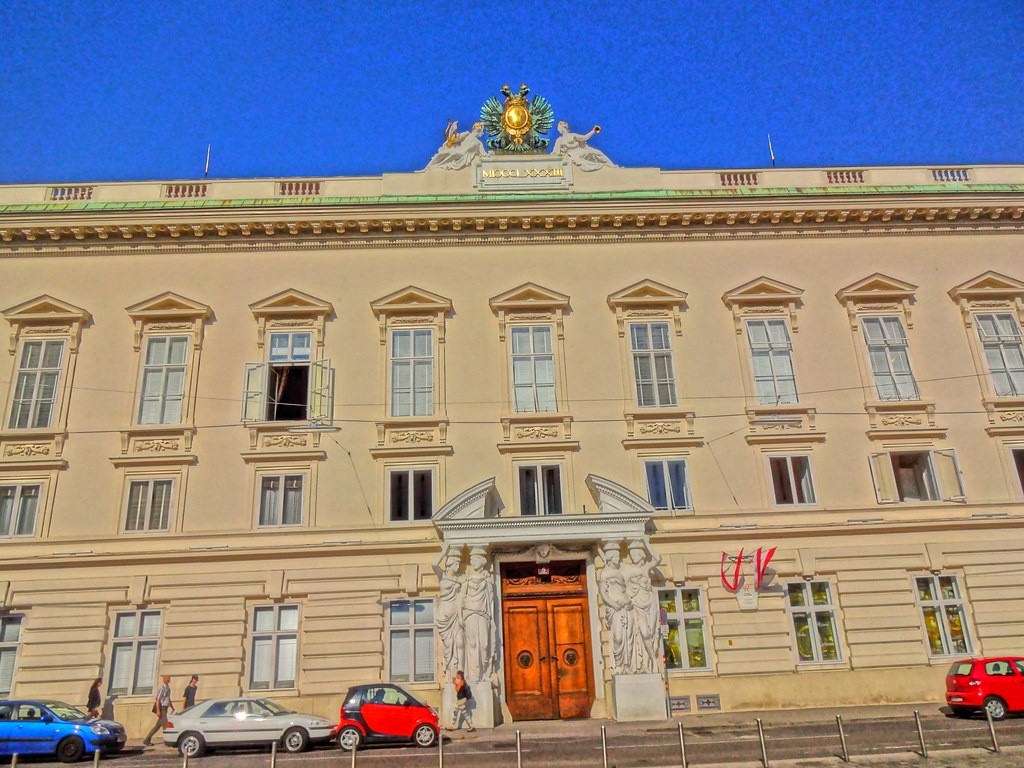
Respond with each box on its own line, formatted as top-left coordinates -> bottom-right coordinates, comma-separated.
465,684 -> 471,699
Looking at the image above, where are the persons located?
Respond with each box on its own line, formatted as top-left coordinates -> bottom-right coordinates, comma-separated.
598,536 -> 662,674
445,670 -> 475,732
414,121 -> 495,174
86,678 -> 102,717
432,542 -> 493,682
143,676 -> 175,746
550,121 -> 619,171
182,675 -> 198,710
371,689 -> 386,704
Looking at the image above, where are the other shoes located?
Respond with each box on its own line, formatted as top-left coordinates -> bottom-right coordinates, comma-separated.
445,726 -> 455,731
143,741 -> 153,746
466,727 -> 475,732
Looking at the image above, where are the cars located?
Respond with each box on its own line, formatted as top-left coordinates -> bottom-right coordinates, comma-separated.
336,684 -> 440,752
0,699 -> 127,763
162,697 -> 339,758
945,657 -> 1024,721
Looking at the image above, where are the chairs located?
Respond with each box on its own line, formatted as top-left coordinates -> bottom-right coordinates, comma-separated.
23,709 -> 39,720
1006,666 -> 1013,675
992,663 -> 1002,675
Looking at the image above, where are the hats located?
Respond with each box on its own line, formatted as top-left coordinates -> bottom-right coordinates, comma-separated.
192,675 -> 198,680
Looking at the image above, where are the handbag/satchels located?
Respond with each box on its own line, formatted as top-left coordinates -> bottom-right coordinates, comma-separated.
152,702 -> 162,714
